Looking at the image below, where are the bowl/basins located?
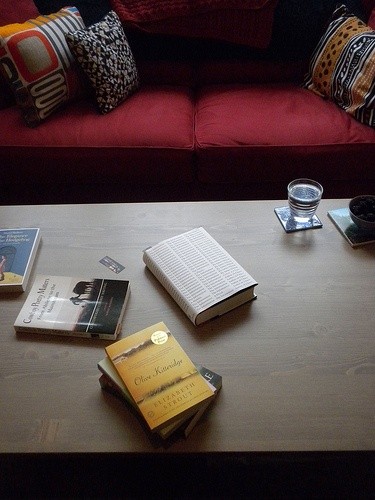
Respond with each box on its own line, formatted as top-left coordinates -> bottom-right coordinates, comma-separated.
349,195 -> 375,231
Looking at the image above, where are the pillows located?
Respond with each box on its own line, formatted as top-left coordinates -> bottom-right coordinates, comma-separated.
298,4 -> 375,130
0,5 -> 86,126
66,11 -> 136,112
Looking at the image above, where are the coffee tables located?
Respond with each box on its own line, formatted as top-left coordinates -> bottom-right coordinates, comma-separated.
0,198 -> 375,457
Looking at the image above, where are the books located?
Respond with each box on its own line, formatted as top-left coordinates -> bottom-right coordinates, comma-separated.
0,227 -> 41,293
97,351 -> 217,440
142,227 -> 260,326
105,321 -> 217,434
99,353 -> 224,438
14,274 -> 131,341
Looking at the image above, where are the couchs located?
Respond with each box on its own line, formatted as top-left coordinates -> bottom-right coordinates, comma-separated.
0,0 -> 375,205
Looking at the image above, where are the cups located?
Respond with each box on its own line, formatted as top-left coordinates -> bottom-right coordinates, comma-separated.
288,179 -> 323,225
